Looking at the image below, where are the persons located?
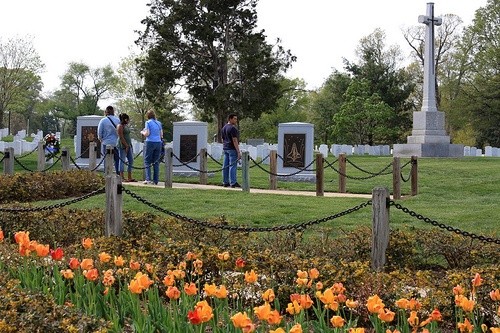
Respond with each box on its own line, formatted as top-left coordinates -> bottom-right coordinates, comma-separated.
117,113 -> 138,182
97,106 -> 120,175
140,110 -> 163,185
222,113 -> 241,188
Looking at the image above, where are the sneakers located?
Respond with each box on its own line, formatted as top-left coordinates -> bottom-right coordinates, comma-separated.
142,180 -> 158,185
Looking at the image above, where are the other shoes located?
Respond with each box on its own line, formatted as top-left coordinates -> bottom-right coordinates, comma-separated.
222,182 -> 230,187
230,182 -> 241,188
119,177 -> 129,182
128,178 -> 138,182
